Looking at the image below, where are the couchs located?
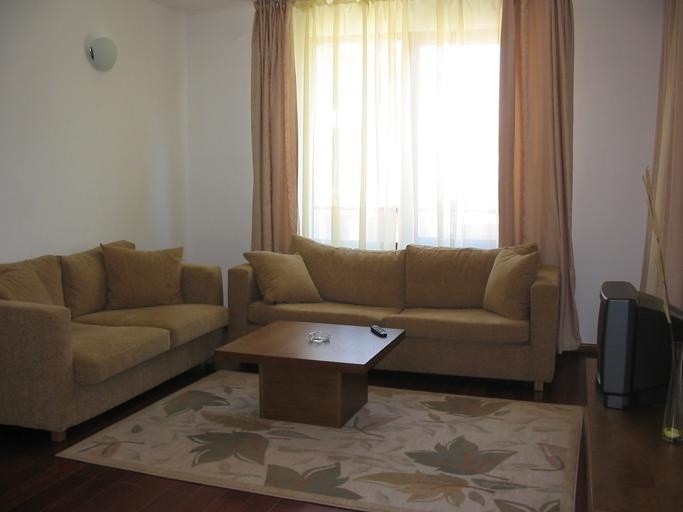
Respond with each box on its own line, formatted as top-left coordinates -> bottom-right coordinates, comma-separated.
226,234 -> 560,392
0,241 -> 230,445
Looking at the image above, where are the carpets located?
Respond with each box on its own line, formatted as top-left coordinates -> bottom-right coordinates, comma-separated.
54,369 -> 584,512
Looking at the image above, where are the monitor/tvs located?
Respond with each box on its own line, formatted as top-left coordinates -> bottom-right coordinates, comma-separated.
595,281 -> 683,409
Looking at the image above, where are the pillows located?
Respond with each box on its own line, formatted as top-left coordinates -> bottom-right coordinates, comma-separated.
481,246 -> 537,321
0,270 -> 51,308
97,242 -> 184,311
242,249 -> 322,306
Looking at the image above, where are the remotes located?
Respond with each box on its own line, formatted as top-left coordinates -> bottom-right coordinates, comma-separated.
372,326 -> 387,337
